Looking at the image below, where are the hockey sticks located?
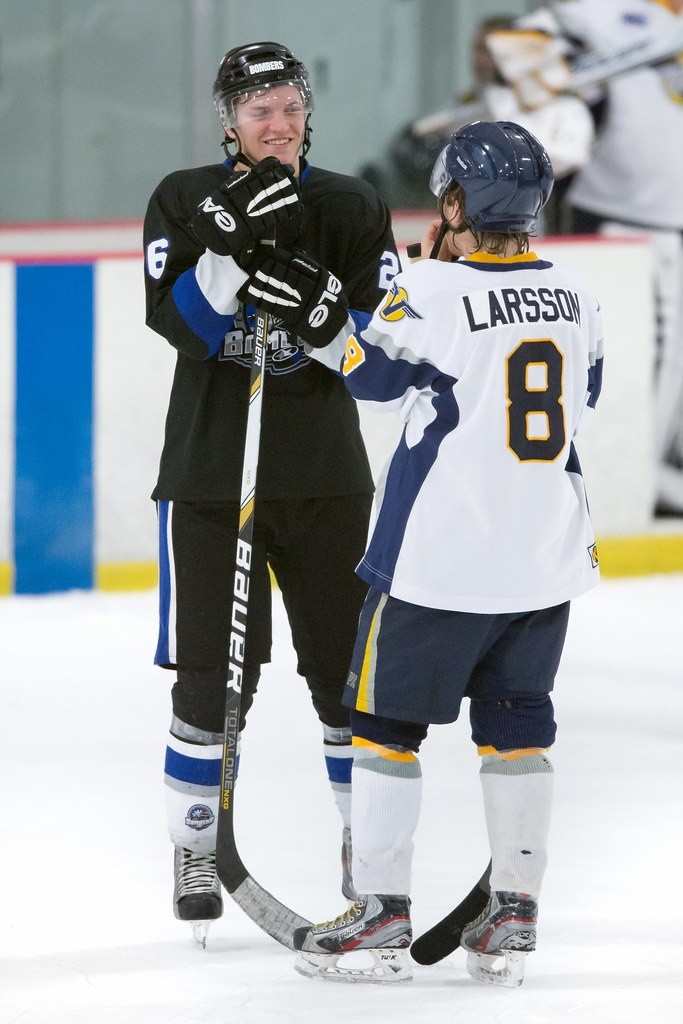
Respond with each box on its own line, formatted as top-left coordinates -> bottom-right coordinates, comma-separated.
213,226 -> 332,951
411,24 -> 682,139
408,855 -> 494,967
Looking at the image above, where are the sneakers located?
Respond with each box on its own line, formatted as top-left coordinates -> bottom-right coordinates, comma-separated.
173,844 -> 223,948
293,895 -> 413,984
459,894 -> 536,989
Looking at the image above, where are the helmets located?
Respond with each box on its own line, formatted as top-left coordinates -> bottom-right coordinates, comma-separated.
428,120 -> 554,234
211,41 -> 316,129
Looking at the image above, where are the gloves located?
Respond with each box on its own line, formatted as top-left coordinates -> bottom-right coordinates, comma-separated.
240,251 -> 350,348
183,156 -> 307,256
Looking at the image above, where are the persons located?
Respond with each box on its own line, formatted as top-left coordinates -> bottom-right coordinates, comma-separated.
138,41 -> 403,919
291,118 -> 612,990
